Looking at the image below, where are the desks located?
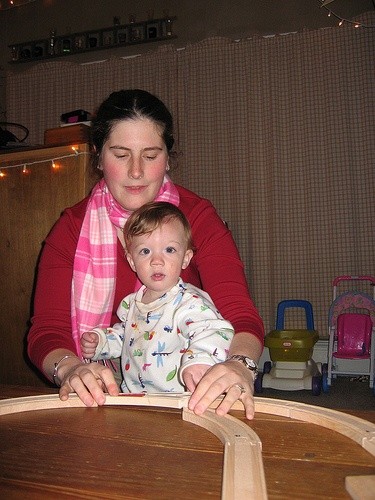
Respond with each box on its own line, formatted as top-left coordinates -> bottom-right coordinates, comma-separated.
0,382 -> 375,500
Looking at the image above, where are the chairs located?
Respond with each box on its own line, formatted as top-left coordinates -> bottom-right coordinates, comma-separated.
322,275 -> 375,394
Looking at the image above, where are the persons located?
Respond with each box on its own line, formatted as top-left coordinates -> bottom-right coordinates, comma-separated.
80,201 -> 235,395
26,88 -> 266,421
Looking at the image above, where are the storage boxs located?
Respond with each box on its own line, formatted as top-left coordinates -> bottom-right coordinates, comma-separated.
264,329 -> 319,363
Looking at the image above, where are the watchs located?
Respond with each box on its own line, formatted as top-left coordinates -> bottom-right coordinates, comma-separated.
226,354 -> 259,383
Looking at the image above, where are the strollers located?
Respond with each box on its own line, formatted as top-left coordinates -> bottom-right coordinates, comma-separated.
322,274 -> 375,393
253,299 -> 328,397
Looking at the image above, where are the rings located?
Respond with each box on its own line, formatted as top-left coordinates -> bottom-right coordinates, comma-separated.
234,383 -> 246,395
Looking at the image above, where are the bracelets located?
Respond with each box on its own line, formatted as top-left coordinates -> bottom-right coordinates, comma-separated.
52,355 -> 84,388
